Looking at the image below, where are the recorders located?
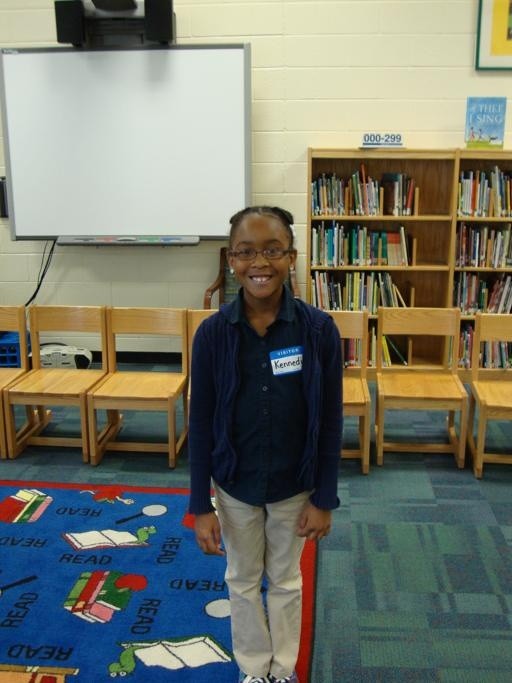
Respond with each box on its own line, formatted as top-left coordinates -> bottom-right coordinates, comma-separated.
28,345 -> 94,370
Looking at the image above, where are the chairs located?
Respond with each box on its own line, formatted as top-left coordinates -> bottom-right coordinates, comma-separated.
87,304 -> 192,468
1,304 -> 39,462
468,311 -> 511,481
320,310 -> 371,474
1,301 -> 124,462
187,305 -> 221,437
373,304 -> 470,474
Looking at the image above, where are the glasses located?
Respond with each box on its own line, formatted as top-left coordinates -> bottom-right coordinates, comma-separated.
228,247 -> 291,259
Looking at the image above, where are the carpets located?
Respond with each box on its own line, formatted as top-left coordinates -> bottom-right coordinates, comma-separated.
1,479 -> 318,683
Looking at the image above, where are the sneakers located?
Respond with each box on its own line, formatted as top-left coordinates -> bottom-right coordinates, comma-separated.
242,673 -> 298,683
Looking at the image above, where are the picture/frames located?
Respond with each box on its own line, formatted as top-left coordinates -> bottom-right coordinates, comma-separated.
474,0 -> 512,71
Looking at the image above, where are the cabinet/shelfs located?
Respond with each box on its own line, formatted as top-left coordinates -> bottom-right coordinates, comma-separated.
306,146 -> 455,386
444,149 -> 512,384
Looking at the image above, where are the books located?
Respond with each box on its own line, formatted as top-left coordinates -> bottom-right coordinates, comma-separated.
311,171 -> 413,368
119,633 -> 233,670
63,571 -> 133,624
0,490 -> 52,524
63,530 -> 151,550
448,164 -> 512,368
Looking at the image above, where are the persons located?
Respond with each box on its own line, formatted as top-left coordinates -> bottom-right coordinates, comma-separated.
189,209 -> 343,682
